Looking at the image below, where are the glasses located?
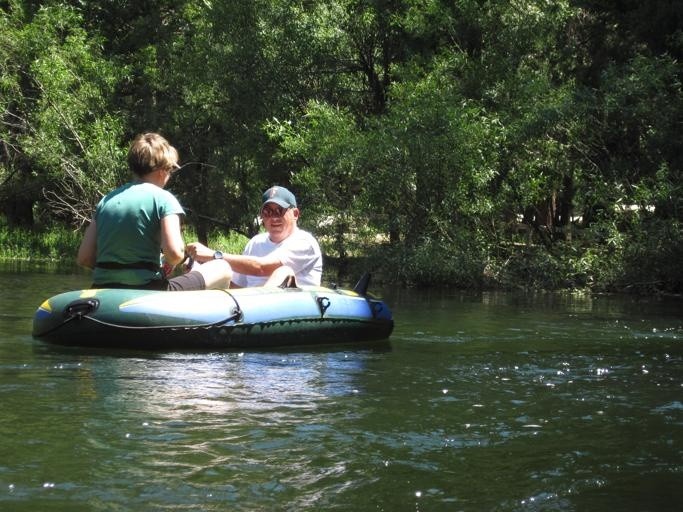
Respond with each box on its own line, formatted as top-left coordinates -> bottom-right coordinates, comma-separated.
262,206 -> 288,217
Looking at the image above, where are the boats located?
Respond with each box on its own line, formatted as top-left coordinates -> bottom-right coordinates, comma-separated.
32,273 -> 393,349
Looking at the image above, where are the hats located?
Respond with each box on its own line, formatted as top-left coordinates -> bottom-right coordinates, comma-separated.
262,186 -> 296,208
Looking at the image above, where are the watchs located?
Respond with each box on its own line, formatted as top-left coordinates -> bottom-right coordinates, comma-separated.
212,250 -> 223,259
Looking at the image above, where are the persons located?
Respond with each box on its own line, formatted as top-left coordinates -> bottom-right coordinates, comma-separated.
179,184 -> 324,289
72,131 -> 233,291
